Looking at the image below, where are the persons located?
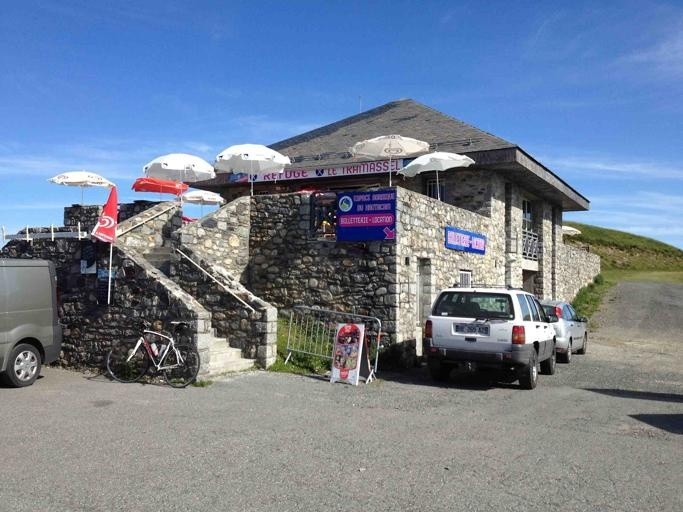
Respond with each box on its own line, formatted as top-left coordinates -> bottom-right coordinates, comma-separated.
139,318 -> 173,374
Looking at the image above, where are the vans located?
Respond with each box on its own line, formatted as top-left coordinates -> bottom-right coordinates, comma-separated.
0,258 -> 62,388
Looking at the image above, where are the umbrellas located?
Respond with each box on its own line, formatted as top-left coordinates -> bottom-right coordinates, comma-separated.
91,187 -> 117,305
172,191 -> 227,220
130,178 -> 188,203
45,170 -> 115,231
213,143 -> 291,196
348,134 -> 429,188
142,153 -> 216,206
395,151 -> 475,201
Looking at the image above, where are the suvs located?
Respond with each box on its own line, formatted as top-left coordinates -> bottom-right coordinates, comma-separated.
422,284 -> 556,392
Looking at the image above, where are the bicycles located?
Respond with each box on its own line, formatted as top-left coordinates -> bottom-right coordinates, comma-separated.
106,317 -> 202,388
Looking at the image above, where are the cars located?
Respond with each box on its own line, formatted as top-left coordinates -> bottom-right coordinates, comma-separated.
539,298 -> 588,362
